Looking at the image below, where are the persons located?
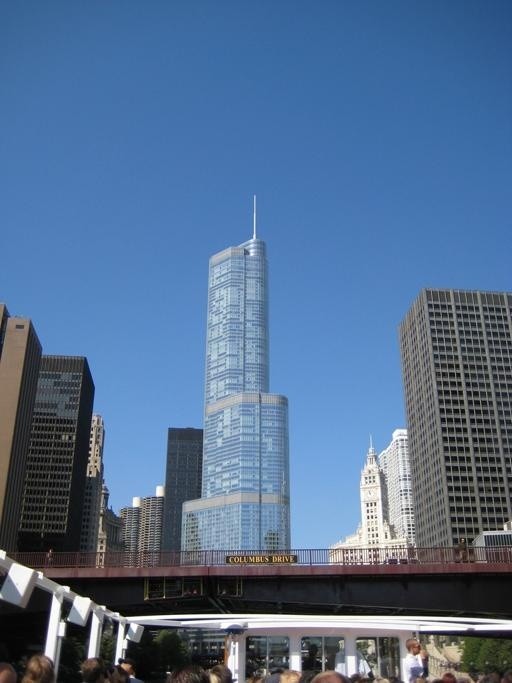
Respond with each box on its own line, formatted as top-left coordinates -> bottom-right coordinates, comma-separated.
458,538 -> 466,564
